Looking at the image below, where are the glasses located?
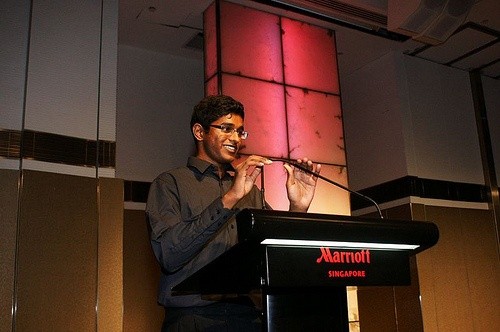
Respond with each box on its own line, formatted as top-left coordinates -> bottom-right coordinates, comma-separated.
210,124 -> 249,139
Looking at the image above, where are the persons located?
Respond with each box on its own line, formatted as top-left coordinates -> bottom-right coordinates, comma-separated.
146,95 -> 321,332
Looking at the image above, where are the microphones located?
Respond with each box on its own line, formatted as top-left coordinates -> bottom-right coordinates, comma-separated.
269,158 -> 384,219
